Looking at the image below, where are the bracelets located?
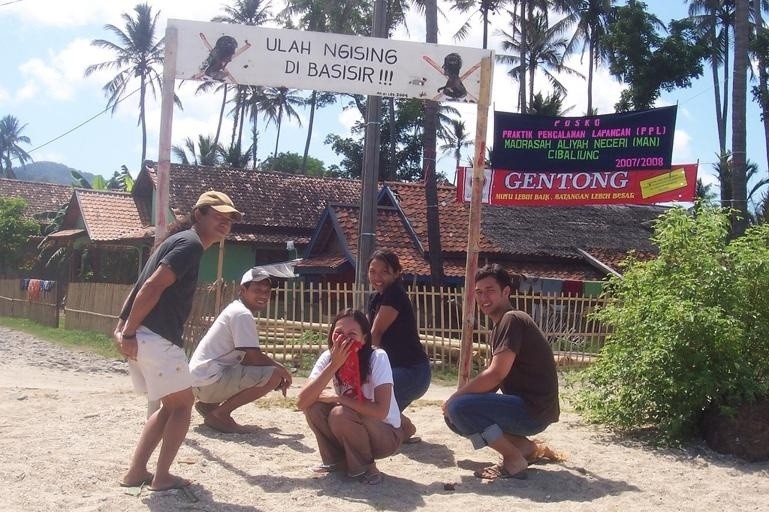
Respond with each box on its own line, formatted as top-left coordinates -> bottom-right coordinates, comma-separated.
121,331 -> 135,339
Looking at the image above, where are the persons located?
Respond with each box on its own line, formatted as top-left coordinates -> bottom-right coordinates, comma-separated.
364,249 -> 431,444
294,308 -> 404,483
442,262 -> 560,478
188,265 -> 293,432
111,189 -> 242,492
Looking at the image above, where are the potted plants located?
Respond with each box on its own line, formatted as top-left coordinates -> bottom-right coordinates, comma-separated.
561,192 -> 769,468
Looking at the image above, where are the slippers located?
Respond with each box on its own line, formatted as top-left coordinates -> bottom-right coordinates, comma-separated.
147,473 -> 194,493
401,435 -> 422,445
310,462 -> 343,475
358,465 -> 383,487
524,449 -> 548,466
118,470 -> 154,488
472,462 -> 531,481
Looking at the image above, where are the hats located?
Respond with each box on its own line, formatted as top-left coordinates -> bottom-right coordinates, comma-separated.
191,189 -> 244,226
240,264 -> 274,287
